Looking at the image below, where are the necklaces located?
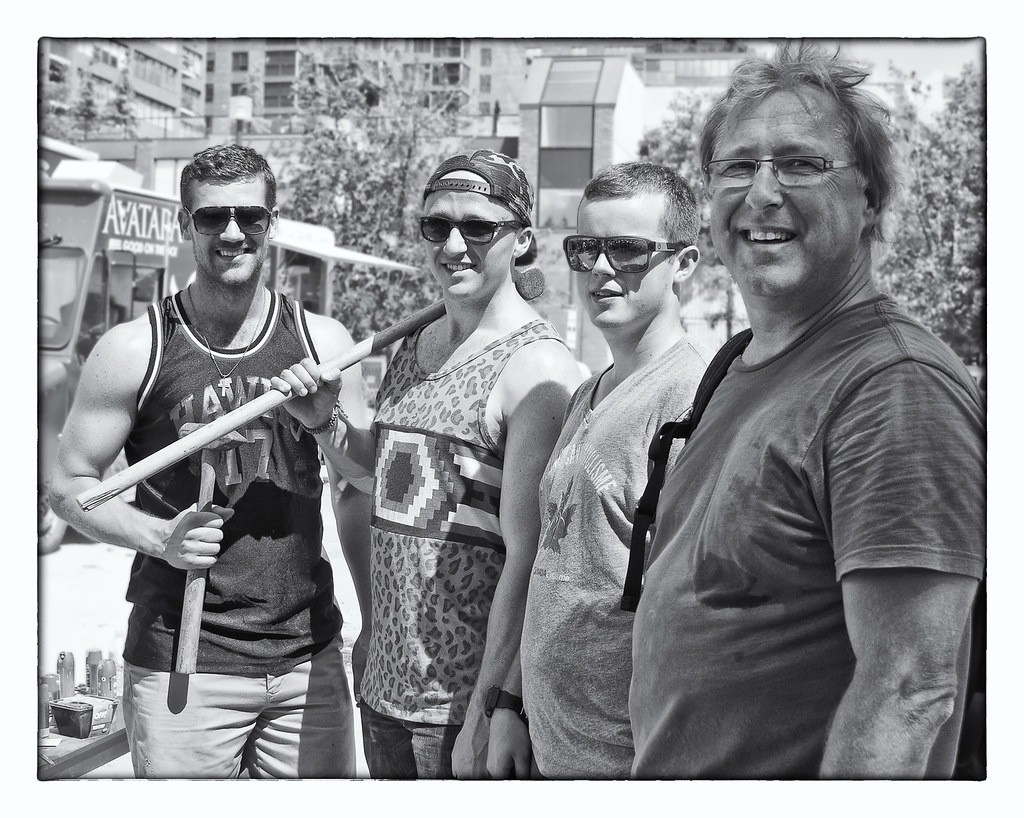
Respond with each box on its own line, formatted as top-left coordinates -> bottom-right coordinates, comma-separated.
188,283 -> 265,397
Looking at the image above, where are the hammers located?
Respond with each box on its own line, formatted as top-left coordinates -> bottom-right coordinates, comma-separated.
175,422 -> 252,675
73,265 -> 548,514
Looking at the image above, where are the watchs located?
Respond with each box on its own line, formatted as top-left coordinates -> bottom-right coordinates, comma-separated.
485,685 -> 529,728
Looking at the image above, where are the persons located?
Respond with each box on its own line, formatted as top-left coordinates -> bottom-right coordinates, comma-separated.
48,147 -> 369,779
269,149 -> 581,781
485,163 -> 719,780
628,39 -> 988,781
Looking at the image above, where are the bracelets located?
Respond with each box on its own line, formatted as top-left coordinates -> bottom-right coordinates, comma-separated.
299,399 -> 349,435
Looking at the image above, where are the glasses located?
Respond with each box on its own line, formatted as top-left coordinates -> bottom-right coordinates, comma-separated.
563,235 -> 685,273
420,216 -> 526,246
703,155 -> 859,188
183,205 -> 272,235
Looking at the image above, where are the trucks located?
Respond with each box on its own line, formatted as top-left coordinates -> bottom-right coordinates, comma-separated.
37,177 -> 420,555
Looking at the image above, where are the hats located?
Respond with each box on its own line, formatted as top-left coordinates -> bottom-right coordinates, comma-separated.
423,149 -> 537,266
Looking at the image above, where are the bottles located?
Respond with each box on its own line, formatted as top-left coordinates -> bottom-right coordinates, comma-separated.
83,649 -> 102,693
37,678 -> 52,735
98,658 -> 117,698
57,652 -> 75,696
